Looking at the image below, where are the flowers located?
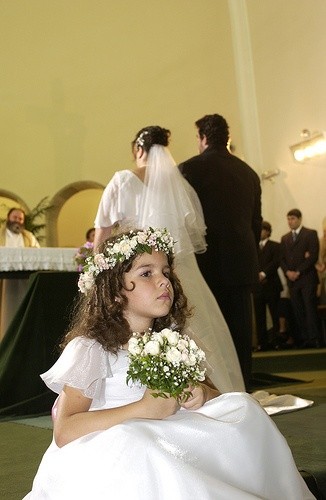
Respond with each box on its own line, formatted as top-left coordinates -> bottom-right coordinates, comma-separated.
78,227 -> 174,294
125,328 -> 206,402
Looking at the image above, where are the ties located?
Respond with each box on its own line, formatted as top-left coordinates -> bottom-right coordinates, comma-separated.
293,232 -> 298,242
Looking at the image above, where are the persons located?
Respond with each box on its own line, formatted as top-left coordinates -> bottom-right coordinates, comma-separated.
170,114 -> 262,386
0,208 -> 40,248
20,226 -> 319,500
252,217 -> 287,352
82,228 -> 95,249
278,209 -> 324,350
93,126 -> 245,397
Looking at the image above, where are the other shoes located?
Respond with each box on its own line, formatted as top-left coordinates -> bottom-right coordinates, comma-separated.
255,343 -> 273,352
284,340 -> 296,348
276,330 -> 289,336
298,340 -> 324,349
